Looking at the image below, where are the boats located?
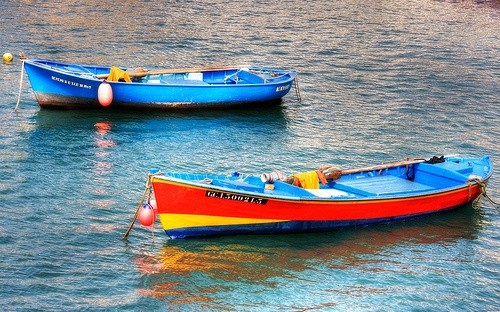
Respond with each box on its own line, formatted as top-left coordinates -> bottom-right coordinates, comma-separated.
3,51 -> 302,114
123,153 -> 500,238
